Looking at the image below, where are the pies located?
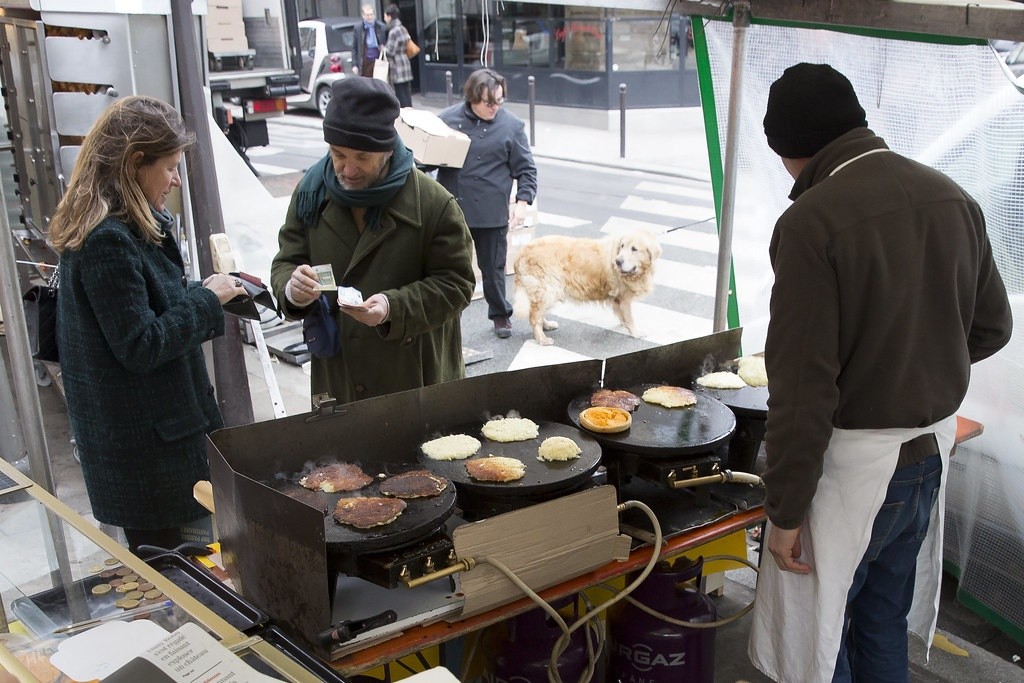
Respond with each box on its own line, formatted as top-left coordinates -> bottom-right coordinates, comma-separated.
421,417 -> 581,482
579,386 -> 698,433
299,464 -> 447,529
697,356 -> 768,389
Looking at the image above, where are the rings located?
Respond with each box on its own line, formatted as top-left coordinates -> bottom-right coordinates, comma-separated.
234,279 -> 243,287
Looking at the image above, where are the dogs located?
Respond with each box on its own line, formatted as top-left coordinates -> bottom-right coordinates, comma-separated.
513,232 -> 664,347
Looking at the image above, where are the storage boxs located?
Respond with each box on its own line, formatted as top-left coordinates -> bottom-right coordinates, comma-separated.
394,115 -> 471,168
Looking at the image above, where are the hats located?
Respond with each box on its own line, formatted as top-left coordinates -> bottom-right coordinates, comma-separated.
763,62 -> 868,159
322,76 -> 401,152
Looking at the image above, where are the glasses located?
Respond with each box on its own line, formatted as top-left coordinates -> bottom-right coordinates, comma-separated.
480,95 -> 507,107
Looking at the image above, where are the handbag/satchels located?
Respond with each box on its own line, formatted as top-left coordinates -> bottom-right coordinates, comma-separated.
23,262 -> 60,363
406,38 -> 420,59
373,48 -> 390,84
302,299 -> 340,358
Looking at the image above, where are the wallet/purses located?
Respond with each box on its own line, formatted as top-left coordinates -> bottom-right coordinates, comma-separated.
221,272 -> 277,322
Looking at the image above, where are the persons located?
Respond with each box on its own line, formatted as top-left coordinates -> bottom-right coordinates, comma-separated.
415,69 -> 537,340
351,5 -> 413,108
745,61 -> 1014,683
44,96 -> 249,560
270,76 -> 476,412
